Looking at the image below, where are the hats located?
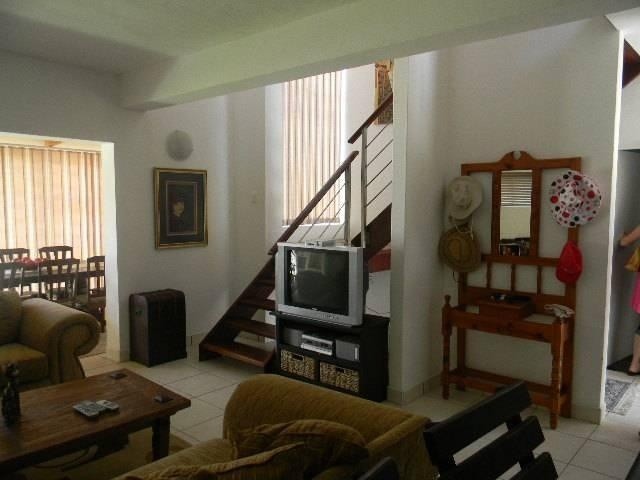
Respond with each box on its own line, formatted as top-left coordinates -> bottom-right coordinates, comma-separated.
557,239 -> 584,285
547,170 -> 602,228
444,176 -> 483,220
438,225 -> 482,274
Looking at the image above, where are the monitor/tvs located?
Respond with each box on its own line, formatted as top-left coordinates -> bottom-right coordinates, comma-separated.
274,242 -> 369,328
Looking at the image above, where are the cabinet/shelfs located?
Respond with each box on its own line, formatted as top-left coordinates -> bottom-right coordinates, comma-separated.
130,289 -> 187,368
270,311 -> 389,402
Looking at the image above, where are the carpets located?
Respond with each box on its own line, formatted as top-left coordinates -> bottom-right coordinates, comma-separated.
607,354 -> 640,375
0,430 -> 192,480
605,379 -> 639,416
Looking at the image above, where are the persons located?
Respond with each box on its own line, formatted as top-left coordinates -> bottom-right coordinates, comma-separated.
618,224 -> 640,375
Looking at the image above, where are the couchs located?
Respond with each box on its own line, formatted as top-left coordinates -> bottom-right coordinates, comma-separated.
112,374 -> 436,480
0,292 -> 101,392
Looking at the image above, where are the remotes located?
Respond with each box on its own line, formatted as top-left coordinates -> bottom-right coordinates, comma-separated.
96,400 -> 120,411
72,404 -> 99,417
80,399 -> 106,414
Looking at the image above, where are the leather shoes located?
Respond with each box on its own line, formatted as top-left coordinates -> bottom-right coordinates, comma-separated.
627,369 -> 639,375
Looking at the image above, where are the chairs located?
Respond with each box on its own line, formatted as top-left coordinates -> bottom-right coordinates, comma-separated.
0,246 -> 105,333
424,381 -> 560,480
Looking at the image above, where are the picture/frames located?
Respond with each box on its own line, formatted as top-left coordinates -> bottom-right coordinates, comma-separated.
154,168 -> 208,249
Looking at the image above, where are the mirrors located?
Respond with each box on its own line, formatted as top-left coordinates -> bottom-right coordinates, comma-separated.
491,151 -> 538,256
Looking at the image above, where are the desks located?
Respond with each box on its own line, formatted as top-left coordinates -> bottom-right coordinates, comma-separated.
442,295 -> 574,430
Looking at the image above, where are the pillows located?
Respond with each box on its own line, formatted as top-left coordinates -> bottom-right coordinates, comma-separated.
127,442 -> 307,480
227,419 -> 370,473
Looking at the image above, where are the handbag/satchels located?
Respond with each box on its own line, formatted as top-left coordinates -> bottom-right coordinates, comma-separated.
552,303 -> 576,319
624,245 -> 640,272
